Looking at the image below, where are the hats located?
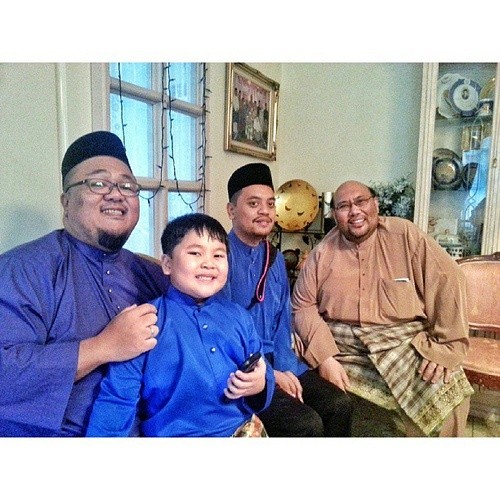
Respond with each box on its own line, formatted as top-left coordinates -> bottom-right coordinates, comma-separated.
227,163 -> 275,201
61,131 -> 132,180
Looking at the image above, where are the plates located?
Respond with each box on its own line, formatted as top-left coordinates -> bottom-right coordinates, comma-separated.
273,179 -> 320,232
432,148 -> 478,191
436,72 -> 462,119
448,78 -> 482,118
478,75 -> 496,101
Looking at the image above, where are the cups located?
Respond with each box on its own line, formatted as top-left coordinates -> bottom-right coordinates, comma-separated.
449,244 -> 463,260
476,98 -> 493,116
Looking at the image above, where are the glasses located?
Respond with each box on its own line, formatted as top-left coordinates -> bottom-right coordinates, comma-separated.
64,178 -> 141,197
331,195 -> 375,211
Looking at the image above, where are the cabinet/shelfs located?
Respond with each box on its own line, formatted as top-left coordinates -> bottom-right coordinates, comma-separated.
270,196 -> 333,290
413,63 -> 500,256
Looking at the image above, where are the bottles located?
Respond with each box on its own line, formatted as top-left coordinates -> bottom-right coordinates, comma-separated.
297,249 -> 310,272
434,228 -> 459,252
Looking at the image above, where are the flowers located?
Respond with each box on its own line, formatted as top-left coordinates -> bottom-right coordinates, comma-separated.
372,170 -> 415,220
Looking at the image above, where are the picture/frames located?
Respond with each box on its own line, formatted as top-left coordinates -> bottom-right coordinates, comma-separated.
223,63 -> 280,161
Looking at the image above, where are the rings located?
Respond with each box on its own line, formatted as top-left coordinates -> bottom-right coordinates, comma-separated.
150,328 -> 154,339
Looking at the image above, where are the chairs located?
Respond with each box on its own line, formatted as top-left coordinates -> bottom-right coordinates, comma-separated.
456,251 -> 500,392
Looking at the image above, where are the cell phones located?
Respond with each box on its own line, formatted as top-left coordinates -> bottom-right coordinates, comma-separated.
220,352 -> 261,404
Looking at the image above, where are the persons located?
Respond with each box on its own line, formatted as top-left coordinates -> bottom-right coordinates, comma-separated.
232,88 -> 269,148
86,213 -> 275,438
223,162 -> 352,438
0,132 -> 170,438
291,181 -> 475,438
